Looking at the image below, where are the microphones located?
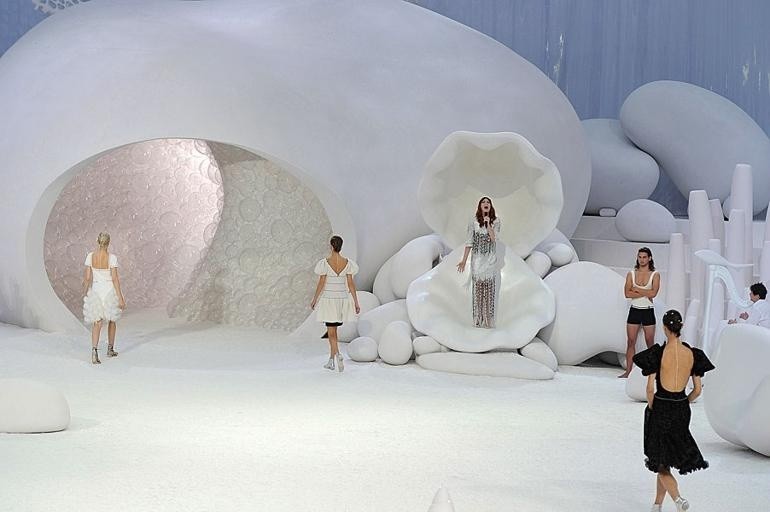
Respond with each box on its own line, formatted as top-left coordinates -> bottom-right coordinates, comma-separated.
485,212 -> 488,229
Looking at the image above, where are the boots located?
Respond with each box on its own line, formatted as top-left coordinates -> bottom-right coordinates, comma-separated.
108,345 -> 117,356
651,503 -> 660,512
92,348 -> 100,363
325,359 -> 334,370
337,352 -> 344,371
674,497 -> 689,512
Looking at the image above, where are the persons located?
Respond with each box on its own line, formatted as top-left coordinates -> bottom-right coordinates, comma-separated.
616,246 -> 662,380
79,230 -> 125,365
308,234 -> 362,374
726,281 -> 769,329
630,310 -> 715,512
456,192 -> 507,331
